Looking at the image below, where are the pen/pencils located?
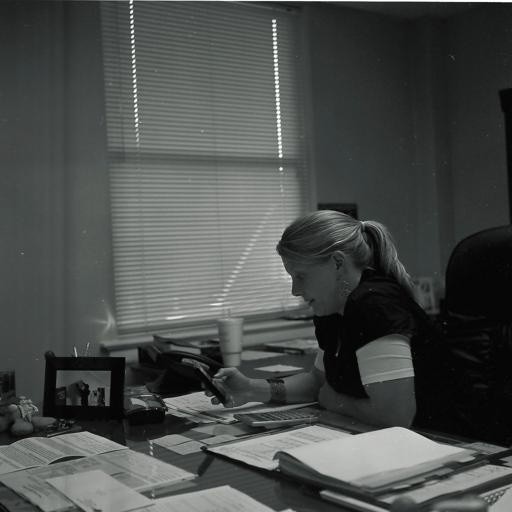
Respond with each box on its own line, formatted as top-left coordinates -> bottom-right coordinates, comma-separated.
74,342 -> 90,357
264,347 -> 304,354
47,426 -> 82,437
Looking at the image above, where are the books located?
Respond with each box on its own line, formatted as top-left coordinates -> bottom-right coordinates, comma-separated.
203,424 -> 510,511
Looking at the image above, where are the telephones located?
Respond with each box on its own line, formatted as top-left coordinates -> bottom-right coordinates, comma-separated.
141,338 -> 210,365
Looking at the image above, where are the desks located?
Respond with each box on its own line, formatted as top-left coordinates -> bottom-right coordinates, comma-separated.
130,335 -> 320,380
0,386 -> 512,512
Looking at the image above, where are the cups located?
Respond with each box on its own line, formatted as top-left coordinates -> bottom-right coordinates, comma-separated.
216,318 -> 244,369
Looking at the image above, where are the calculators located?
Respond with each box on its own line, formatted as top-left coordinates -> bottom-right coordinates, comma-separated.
233,408 -> 319,430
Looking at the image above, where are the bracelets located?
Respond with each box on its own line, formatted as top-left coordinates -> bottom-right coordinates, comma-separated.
264,378 -> 286,406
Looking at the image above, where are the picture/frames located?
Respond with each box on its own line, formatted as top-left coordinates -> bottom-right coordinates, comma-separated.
43,356 -> 125,418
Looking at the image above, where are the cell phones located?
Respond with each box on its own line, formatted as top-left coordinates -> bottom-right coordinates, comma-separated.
191,360 -> 231,404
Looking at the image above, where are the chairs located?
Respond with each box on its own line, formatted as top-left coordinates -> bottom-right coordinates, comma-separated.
435,225 -> 512,319
432,318 -> 512,449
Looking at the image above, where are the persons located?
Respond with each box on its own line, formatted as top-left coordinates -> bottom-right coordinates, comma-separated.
203,211 -> 486,441
66,380 -> 104,407
